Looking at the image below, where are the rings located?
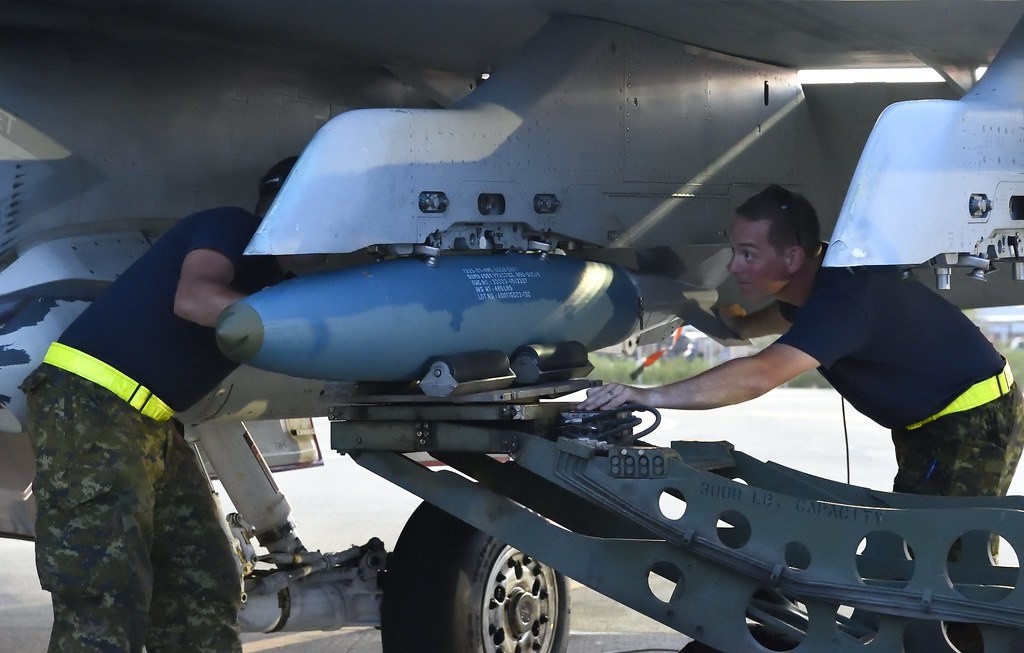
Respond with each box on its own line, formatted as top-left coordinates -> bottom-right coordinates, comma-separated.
607,391 -> 615,398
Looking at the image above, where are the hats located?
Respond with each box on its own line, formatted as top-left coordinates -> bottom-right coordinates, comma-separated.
260,156 -> 297,200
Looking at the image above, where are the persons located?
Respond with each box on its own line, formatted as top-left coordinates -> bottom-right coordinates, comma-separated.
577,185 -> 1024,653
20,152 -> 327,653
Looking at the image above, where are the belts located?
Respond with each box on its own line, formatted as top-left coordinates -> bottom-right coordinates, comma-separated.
905,354 -> 1014,430
42,341 -> 174,422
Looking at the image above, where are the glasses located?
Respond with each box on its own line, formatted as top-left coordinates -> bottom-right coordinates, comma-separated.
759,184 -> 801,246
